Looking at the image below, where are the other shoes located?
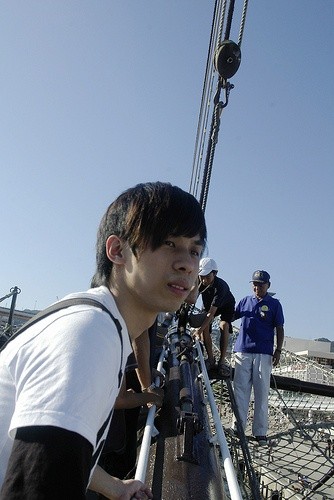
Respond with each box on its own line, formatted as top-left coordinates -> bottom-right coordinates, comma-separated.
256,436 -> 267,444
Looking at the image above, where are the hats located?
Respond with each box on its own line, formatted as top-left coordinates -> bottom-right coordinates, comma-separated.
197,258 -> 219,276
248,270 -> 271,284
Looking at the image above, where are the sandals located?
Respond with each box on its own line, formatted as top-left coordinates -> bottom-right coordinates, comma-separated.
217,360 -> 231,377
203,357 -> 216,371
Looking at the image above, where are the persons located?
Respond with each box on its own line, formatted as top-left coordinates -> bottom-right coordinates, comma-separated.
86,312 -> 167,500
230,270 -> 284,446
0,182 -> 207,500
186,257 -> 235,377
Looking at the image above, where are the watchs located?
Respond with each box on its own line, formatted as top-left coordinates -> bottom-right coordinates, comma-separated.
142,385 -> 156,392
275,349 -> 281,354
